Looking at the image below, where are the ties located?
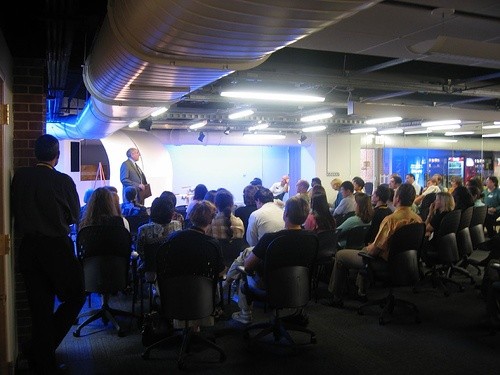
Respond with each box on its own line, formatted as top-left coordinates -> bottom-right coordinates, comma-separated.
135,163 -> 142,175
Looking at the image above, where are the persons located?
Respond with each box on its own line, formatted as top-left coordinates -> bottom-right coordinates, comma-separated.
270,172 -> 500,308
120,148 -> 148,205
12,135 -> 86,375
77,177 -> 310,325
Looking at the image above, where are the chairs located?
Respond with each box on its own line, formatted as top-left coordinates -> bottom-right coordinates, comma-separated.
73,193 -> 500,369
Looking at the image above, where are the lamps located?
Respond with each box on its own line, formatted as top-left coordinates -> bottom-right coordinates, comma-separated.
298,136 -> 306,144
198,131 -> 205,142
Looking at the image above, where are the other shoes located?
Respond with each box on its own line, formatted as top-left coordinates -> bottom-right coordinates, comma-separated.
213,309 -> 223,318
320,297 -> 340,307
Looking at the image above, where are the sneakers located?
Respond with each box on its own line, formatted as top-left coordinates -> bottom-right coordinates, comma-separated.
232,309 -> 252,323
